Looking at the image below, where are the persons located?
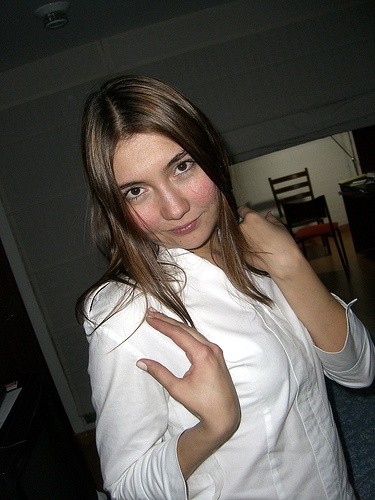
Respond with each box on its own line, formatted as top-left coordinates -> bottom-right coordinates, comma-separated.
76,73 -> 375,500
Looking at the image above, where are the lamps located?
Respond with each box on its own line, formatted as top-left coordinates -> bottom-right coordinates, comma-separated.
34,0 -> 70,29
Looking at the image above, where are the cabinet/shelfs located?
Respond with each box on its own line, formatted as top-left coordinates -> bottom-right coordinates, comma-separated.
338,175 -> 375,254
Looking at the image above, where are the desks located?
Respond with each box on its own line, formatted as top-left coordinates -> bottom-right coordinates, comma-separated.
0,356 -> 75,499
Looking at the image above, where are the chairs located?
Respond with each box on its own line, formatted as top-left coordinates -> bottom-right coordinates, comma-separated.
279,194 -> 350,283
268,167 -> 326,247
